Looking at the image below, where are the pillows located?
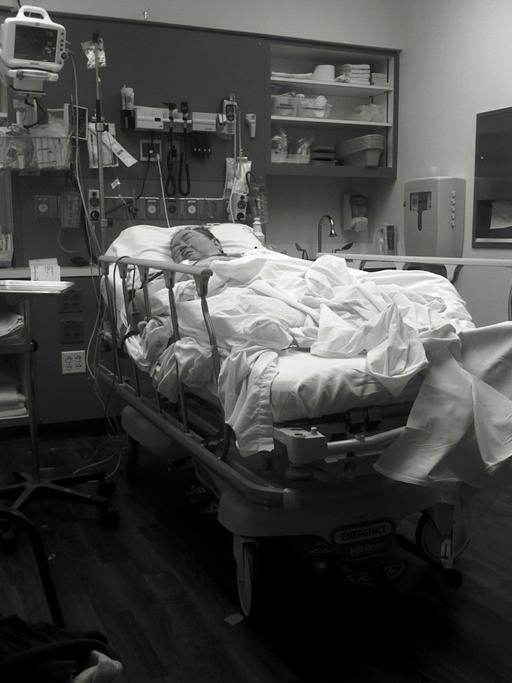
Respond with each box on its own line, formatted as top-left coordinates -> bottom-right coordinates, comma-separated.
105,223 -> 264,294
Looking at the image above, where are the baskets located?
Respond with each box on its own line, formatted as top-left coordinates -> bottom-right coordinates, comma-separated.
0,137 -> 71,170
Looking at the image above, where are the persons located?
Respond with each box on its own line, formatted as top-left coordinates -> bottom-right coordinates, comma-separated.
134,225 -> 245,310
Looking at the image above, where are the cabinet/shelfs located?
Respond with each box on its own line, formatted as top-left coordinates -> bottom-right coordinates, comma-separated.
266,41 -> 395,173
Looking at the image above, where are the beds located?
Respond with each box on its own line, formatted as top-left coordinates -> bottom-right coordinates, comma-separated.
90,218 -> 511,626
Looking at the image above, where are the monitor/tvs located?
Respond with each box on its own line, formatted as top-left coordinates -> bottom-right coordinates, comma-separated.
0,17 -> 66,73
